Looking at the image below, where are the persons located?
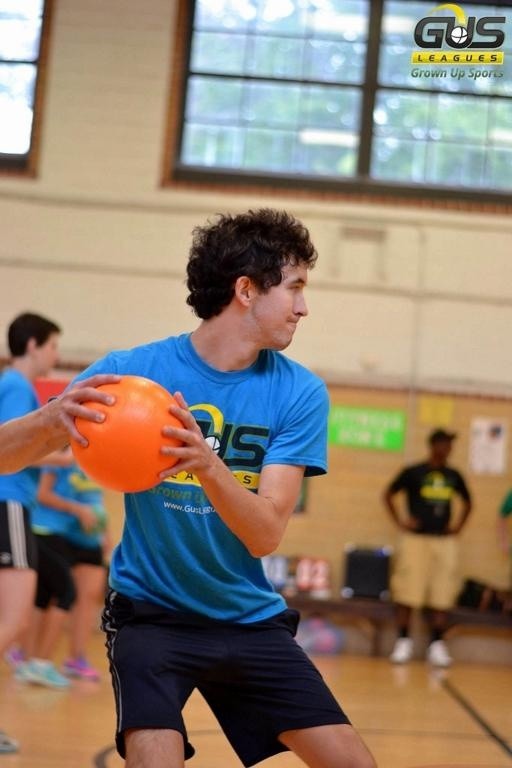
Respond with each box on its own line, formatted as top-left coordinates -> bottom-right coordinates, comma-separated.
497,489 -> 512,546
2,311 -> 63,754
18,466 -> 109,689
384,429 -> 472,668
1,208 -> 376,768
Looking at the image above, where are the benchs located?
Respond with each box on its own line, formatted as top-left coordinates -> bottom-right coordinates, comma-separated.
284,595 -> 449,657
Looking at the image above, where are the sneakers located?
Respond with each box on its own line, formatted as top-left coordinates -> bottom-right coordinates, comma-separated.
16,662 -> 69,687
0,732 -> 19,752
5,641 -> 26,671
427,639 -> 451,665
390,638 -> 412,662
63,655 -> 100,678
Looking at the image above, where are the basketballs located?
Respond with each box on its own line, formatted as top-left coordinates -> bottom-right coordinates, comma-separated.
73,374 -> 189,492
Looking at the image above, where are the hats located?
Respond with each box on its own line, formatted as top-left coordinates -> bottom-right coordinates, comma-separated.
431,431 -> 455,443
